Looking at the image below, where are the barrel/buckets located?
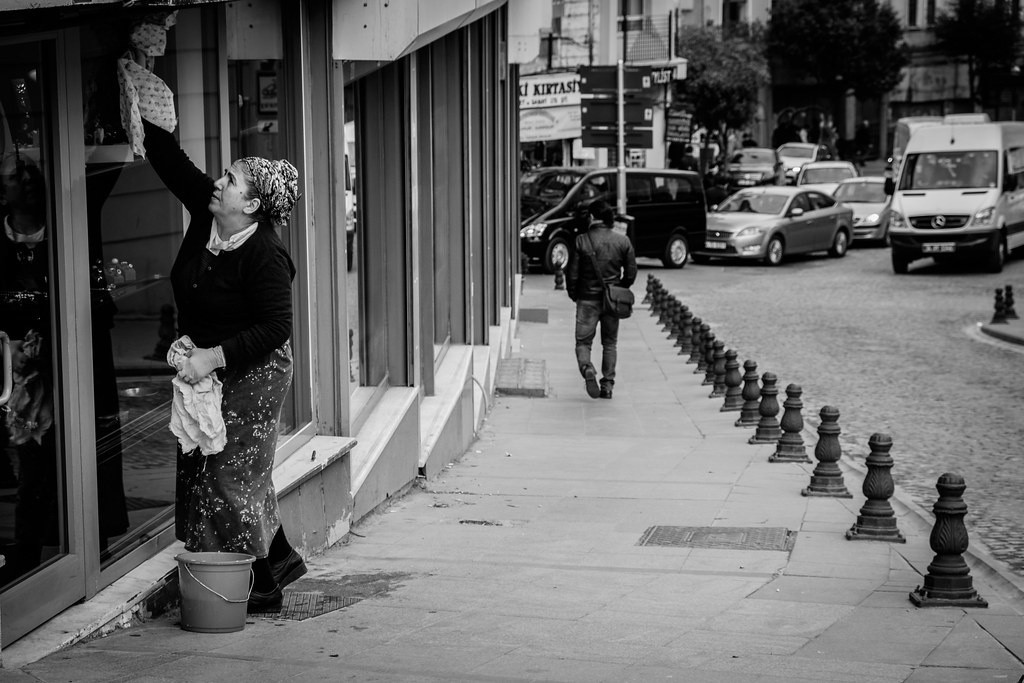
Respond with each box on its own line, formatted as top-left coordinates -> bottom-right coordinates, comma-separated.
174,552 -> 256,634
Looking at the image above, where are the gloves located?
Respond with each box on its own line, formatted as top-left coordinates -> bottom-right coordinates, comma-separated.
176,345 -> 226,384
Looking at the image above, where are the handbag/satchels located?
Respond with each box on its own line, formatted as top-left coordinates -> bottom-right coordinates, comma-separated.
603,284 -> 634,318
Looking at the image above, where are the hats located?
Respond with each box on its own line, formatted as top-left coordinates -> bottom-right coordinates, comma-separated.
586,200 -> 609,217
243,156 -> 302,225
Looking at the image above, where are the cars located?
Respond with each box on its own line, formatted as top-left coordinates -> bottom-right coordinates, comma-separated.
775,142 -> 831,189
830,175 -> 897,246
793,161 -> 859,198
687,186 -> 855,267
519,168 -> 598,218
723,148 -> 775,193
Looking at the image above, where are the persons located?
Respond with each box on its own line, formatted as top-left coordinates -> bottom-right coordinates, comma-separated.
123,41 -> 307,616
0,61 -> 129,588
566,200 -> 638,398
668,133 -> 729,212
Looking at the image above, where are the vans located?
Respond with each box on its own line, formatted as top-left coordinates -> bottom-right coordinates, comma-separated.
885,114 -> 1024,275
516,166 -> 709,275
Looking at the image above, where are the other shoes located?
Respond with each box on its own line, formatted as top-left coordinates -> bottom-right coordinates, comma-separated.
600,389 -> 611,399
271,547 -> 308,590
585,368 -> 600,398
248,583 -> 282,614
